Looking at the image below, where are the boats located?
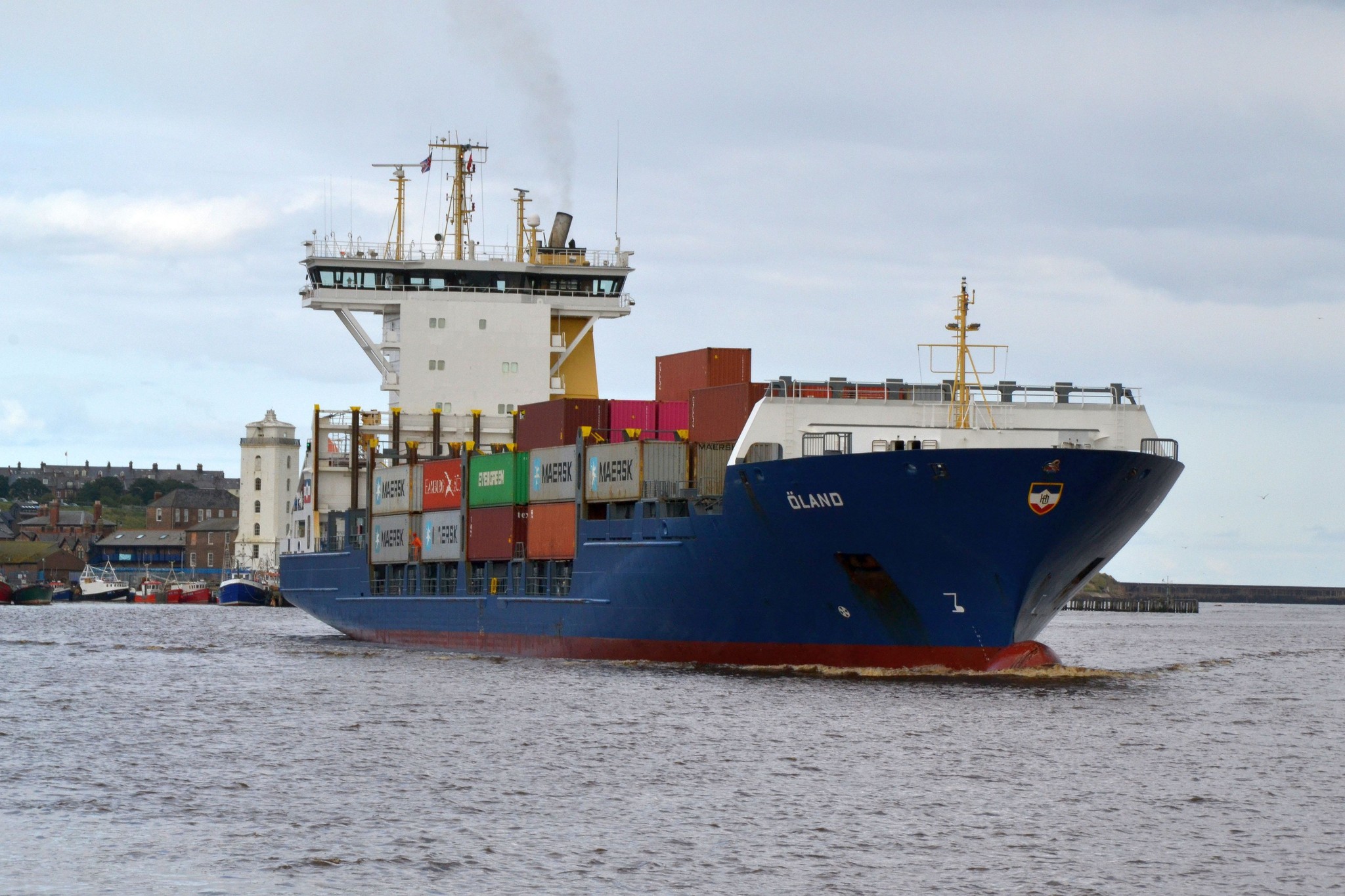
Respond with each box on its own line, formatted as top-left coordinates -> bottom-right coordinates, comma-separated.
170,560 -> 210,604
127,553 -> 183,605
47,567 -> 71,601
278,124 -> 1191,676
0,572 -> 14,605
215,533 -> 270,606
77,555 -> 129,601
8,583 -> 55,606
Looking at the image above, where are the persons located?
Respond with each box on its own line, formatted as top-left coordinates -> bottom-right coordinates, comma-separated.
408,533 -> 421,558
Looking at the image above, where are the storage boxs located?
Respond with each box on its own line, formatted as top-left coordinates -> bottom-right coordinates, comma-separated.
372,348 -> 944,565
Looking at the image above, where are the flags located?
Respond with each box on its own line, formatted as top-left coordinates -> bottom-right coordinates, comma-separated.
420,152 -> 432,174
466,152 -> 472,175
